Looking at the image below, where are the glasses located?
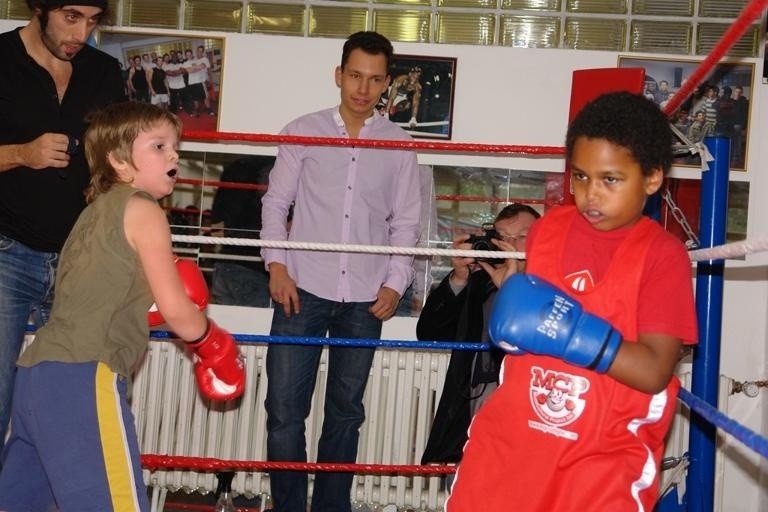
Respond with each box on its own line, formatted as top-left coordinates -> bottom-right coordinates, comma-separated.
501,233 -> 526,242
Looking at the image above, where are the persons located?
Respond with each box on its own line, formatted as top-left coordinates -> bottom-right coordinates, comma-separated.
641,74 -> 746,160
382,67 -> 421,130
441,92 -> 700,511
2,103 -> 246,511
122,46 -> 216,123
0,2 -> 125,466
260,31 -> 421,511
416,204 -> 542,485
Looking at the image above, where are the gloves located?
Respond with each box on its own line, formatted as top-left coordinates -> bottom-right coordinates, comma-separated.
147,255 -> 209,327
182,319 -> 247,402
488,272 -> 623,374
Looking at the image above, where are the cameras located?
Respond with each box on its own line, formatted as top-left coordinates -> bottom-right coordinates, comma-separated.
465,223 -> 505,264
